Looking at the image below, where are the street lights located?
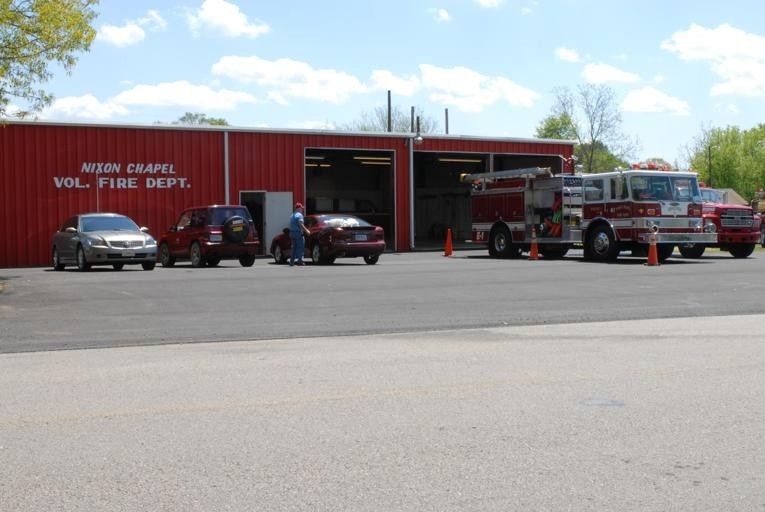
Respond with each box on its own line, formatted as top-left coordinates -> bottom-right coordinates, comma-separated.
707,145 -> 721,186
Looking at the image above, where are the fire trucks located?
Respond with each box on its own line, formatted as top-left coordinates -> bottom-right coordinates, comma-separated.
459,155 -> 708,264
749,184 -> 765,247
671,181 -> 763,258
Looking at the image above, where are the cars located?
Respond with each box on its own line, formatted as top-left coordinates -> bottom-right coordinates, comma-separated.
47,209 -> 162,273
267,211 -> 388,262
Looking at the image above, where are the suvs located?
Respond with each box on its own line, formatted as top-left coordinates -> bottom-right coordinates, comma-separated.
157,199 -> 264,272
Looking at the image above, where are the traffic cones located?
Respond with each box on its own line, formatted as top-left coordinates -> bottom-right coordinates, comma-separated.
526,226 -> 542,261
643,229 -> 663,267
440,228 -> 454,257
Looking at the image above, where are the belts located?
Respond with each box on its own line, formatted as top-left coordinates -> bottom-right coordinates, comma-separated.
289,231 -> 303,232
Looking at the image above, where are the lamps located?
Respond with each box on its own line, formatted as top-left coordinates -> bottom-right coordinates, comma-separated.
404,130 -> 425,148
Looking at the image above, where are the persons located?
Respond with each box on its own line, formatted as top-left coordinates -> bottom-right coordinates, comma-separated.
288,202 -> 311,266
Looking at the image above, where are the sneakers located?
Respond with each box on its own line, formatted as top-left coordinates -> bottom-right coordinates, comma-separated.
298,260 -> 306,265
289,260 -> 295,266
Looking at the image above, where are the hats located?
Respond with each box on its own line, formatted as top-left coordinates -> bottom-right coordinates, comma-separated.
295,202 -> 305,208
